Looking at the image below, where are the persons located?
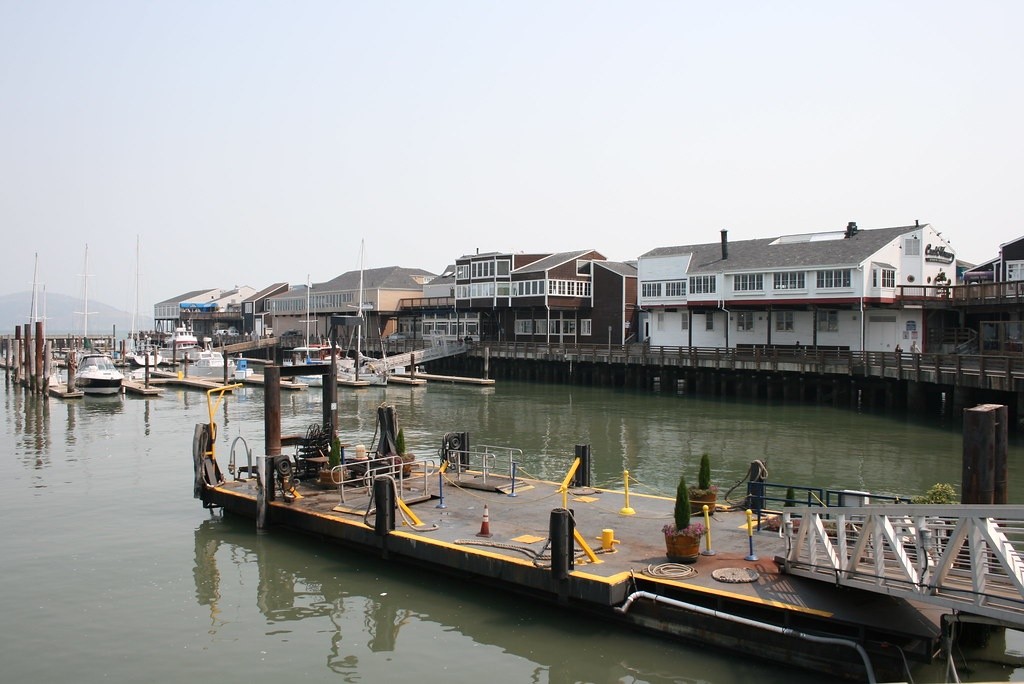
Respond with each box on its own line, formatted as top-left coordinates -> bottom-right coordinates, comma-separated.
793,341 -> 802,358
910,341 -> 922,369
458,336 -> 473,346
895,344 -> 903,365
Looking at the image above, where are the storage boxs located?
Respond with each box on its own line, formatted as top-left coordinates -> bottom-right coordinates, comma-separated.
395,367 -> 405,374
234,371 -> 246,379
246,371 -> 252,377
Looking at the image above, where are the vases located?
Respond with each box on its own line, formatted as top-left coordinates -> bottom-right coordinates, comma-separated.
395,462 -> 411,480
320,472 -> 339,490
688,494 -> 718,517
665,534 -> 702,565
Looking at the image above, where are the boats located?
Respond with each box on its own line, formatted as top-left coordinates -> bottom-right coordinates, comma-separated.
28,235 -> 393,397
84,394 -> 125,421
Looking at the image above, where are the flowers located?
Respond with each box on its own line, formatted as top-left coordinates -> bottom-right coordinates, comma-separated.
318,396 -> 373,475
661,476 -> 709,546
687,453 -> 720,497
387,428 -> 415,464
